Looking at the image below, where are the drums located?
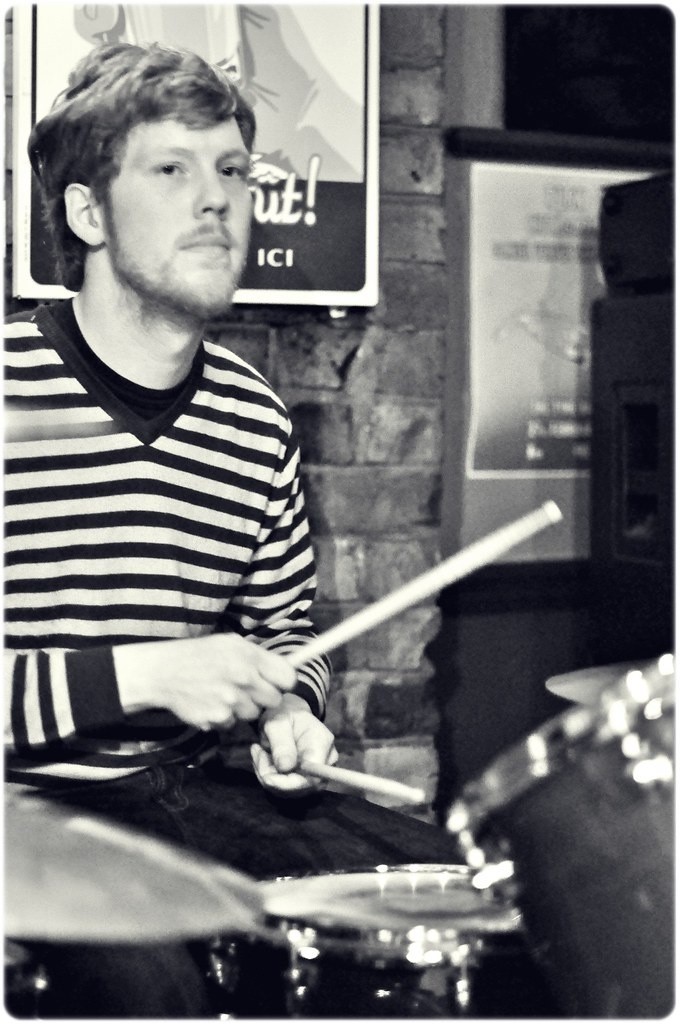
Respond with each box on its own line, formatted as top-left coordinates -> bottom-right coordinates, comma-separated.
431,629 -> 680,1018
251,858 -> 523,1021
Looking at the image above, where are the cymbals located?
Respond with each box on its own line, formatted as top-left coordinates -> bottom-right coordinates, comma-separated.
0,781 -> 268,944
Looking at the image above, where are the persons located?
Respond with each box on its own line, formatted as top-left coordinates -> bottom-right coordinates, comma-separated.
4,45 -> 468,1019
